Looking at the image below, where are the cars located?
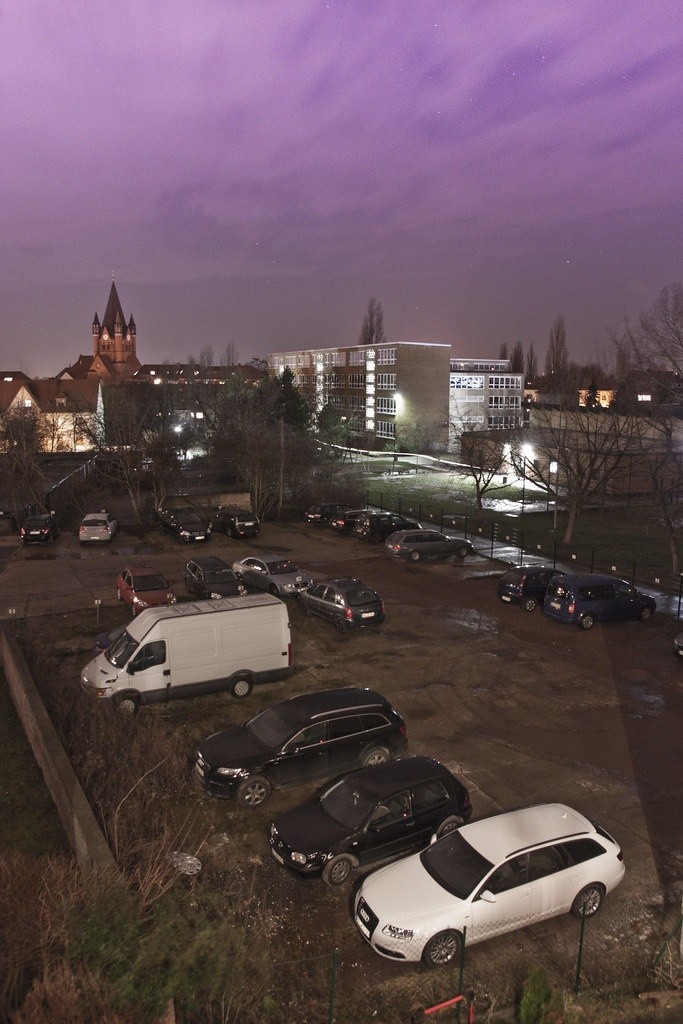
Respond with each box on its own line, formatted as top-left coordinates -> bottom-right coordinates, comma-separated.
232,554 -> 314,599
498,565 -> 567,612
156,507 -> 211,545
79,513 -> 118,545
117,568 -> 178,617
329,509 -> 376,532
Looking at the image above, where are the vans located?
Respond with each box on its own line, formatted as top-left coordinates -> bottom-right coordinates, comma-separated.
80,592 -> 292,716
544,574 -> 657,631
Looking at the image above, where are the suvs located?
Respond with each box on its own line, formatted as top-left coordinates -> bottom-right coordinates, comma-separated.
269,755 -> 474,889
354,512 -> 421,544
297,578 -> 385,633
21,513 -> 58,545
384,529 -> 473,562
192,685 -> 407,810
354,803 -> 625,968
184,556 -> 247,600
209,510 -> 260,540
304,502 -> 356,527
